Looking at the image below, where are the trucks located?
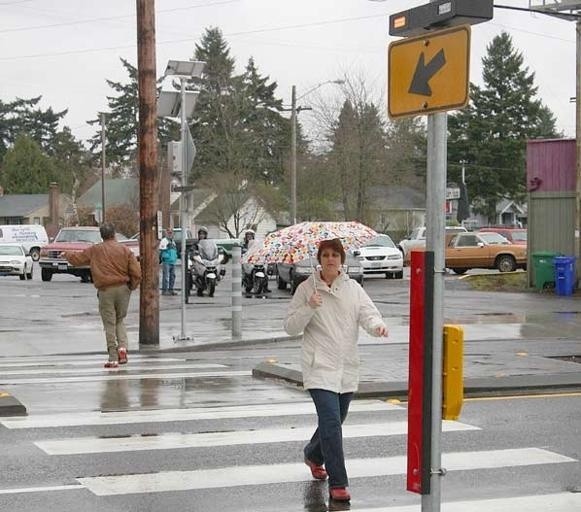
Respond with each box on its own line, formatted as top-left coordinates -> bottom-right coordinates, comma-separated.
0,224 -> 49,261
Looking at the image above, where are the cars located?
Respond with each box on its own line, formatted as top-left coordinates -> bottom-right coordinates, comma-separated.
277,248 -> 363,296
0,241 -> 33,280
443,230 -> 527,272
349,235 -> 404,279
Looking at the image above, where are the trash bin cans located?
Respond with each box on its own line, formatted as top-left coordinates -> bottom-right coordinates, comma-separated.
532,251 -> 562,289
552,255 -> 576,296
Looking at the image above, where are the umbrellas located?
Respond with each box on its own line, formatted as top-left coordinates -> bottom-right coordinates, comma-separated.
239,220 -> 380,294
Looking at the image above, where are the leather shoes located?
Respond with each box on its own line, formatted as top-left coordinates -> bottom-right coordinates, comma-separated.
262,288 -> 271,293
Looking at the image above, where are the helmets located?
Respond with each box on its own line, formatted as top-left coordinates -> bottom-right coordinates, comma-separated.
197,226 -> 208,235
243,229 -> 255,244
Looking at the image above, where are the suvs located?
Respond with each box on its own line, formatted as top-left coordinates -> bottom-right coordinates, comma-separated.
399,226 -> 468,263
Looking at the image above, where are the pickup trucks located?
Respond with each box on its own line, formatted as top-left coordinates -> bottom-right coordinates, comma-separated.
131,228 -> 241,264
37,226 -> 139,282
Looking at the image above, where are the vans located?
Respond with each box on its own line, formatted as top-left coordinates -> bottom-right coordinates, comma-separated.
480,228 -> 527,249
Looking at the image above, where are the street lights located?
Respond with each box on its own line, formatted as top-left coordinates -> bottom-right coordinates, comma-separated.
288,78 -> 344,222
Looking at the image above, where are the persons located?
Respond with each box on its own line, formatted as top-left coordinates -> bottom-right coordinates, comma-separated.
158,228 -> 178,296
238,234 -> 272,293
60,222 -> 142,368
189,231 -> 213,296
283,237 -> 387,501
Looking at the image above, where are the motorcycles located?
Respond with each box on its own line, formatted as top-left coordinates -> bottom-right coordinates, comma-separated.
237,241 -> 271,297
186,241 -> 229,297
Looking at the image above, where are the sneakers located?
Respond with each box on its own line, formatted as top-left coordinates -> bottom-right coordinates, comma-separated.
103,360 -> 118,368
169,290 -> 177,296
117,350 -> 127,365
328,487 -> 351,502
161,291 -> 171,296
302,455 -> 327,480
